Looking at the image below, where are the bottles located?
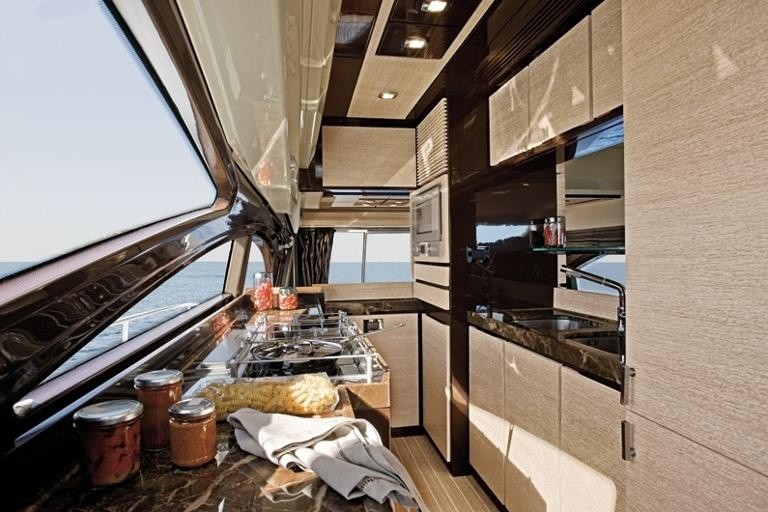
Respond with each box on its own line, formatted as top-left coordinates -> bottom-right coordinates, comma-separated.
252,271 -> 275,309
528,215 -> 565,249
279,287 -> 298,309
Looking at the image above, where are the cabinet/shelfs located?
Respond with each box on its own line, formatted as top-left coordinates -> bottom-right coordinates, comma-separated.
505,337 -> 558,512
591,0 -> 622,116
466,326 -> 506,512
558,359 -> 624,512
623,408 -> 766,511
624,2 -> 767,480
323,300 -> 420,436
489,67 -> 528,169
529,16 -> 592,153
422,315 -> 465,478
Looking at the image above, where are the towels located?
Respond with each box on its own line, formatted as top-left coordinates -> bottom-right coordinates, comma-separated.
226,407 -> 415,510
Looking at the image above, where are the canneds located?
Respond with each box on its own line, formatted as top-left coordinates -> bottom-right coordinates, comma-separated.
529,217 -> 566,247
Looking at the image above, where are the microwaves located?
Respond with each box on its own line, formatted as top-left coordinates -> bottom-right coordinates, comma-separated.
409,184 -> 443,242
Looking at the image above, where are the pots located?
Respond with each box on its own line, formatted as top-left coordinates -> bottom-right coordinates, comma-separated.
250,322 -> 406,370
